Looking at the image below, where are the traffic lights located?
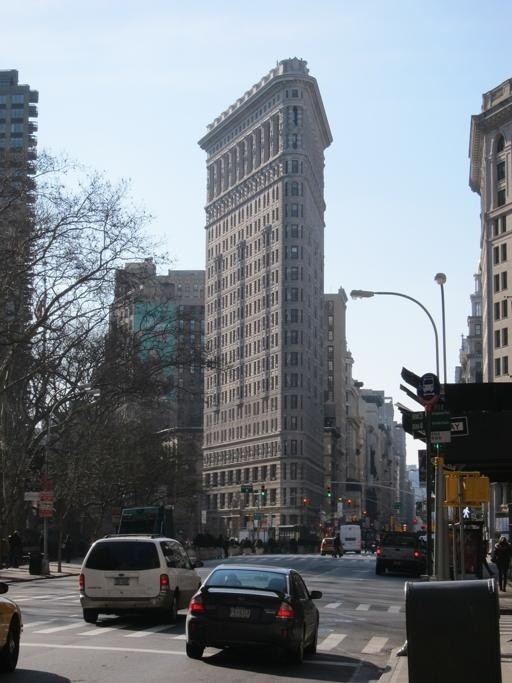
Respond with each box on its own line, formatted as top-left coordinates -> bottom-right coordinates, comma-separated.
303,496 -> 309,504
462,506 -> 471,520
260,488 -> 268,498
327,487 -> 352,505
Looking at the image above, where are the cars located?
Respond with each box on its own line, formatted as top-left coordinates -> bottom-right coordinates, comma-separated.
0,580 -> 22,671
375,531 -> 427,576
79,533 -> 322,665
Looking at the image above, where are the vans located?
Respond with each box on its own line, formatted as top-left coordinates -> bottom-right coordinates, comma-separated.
321,525 -> 361,555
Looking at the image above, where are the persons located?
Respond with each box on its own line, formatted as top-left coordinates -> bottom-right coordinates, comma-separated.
332,531 -> 345,557
222,536 -> 229,559
63,535 -> 74,563
475,539 -> 495,578
39,529 -> 50,553
8,530 -> 24,568
171,526 -> 187,545
490,534 -> 511,592
369,541 -> 377,554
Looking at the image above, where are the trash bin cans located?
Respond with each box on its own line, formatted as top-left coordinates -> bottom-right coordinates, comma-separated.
29,551 -> 42,575
405,579 -> 502,683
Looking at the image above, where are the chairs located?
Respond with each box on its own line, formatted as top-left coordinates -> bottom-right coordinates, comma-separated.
224,572 -> 284,592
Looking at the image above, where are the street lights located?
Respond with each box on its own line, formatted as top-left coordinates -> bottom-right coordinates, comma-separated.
41,383 -> 101,575
350,273 -> 447,382
